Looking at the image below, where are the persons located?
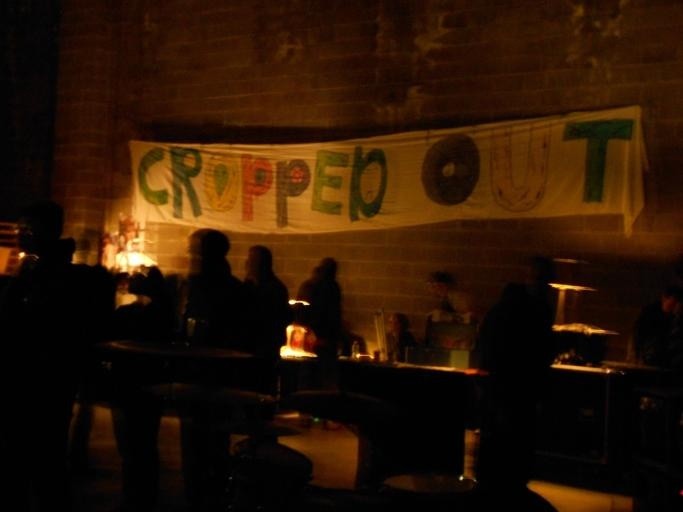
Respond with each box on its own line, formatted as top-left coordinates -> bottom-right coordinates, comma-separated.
625,284 -> 683,374
476,255 -> 558,494
417,271 -> 460,363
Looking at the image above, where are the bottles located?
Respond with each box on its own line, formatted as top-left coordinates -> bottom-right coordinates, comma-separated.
351,341 -> 360,361
